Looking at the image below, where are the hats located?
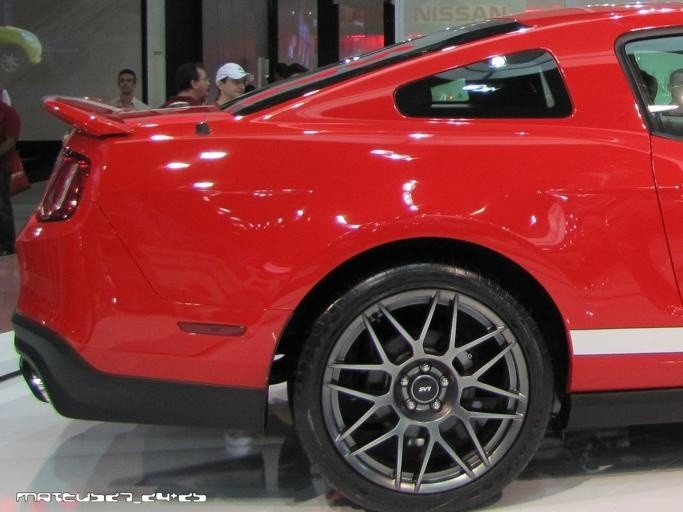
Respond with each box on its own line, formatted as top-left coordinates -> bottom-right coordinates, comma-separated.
216,63 -> 254,83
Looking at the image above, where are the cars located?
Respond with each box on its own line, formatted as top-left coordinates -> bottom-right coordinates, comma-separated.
11,3 -> 682,510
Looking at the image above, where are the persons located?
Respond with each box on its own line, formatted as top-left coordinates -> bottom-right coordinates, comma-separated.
244,61 -> 306,94
104,70 -> 151,111
211,62 -> 255,108
156,61 -> 212,109
659,68 -> 683,118
